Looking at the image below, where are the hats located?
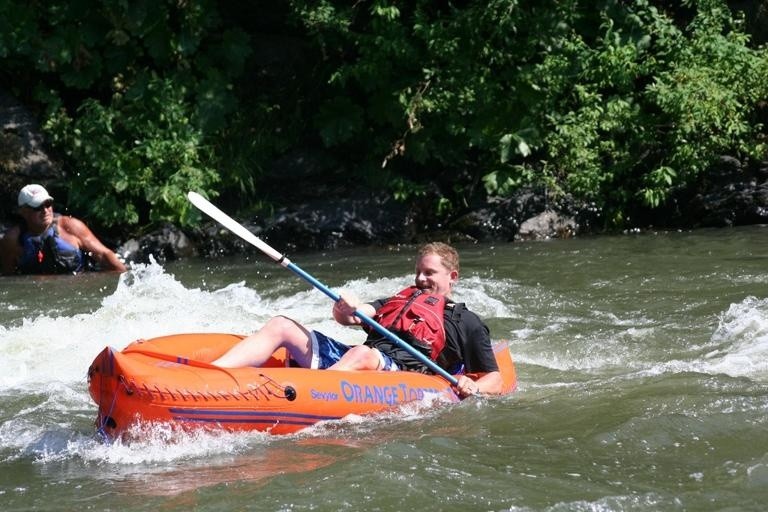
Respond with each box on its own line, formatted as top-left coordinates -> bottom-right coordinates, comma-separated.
18,184 -> 54,208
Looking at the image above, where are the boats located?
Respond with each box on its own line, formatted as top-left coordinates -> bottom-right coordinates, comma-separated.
87,331 -> 519,444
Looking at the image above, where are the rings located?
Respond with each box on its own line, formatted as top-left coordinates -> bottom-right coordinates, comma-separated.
467,387 -> 473,391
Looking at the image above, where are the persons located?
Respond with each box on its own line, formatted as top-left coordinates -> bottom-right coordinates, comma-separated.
210,240 -> 505,400
0,183 -> 129,276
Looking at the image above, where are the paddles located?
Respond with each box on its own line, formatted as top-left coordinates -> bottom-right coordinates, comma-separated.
187,190 -> 458,387
